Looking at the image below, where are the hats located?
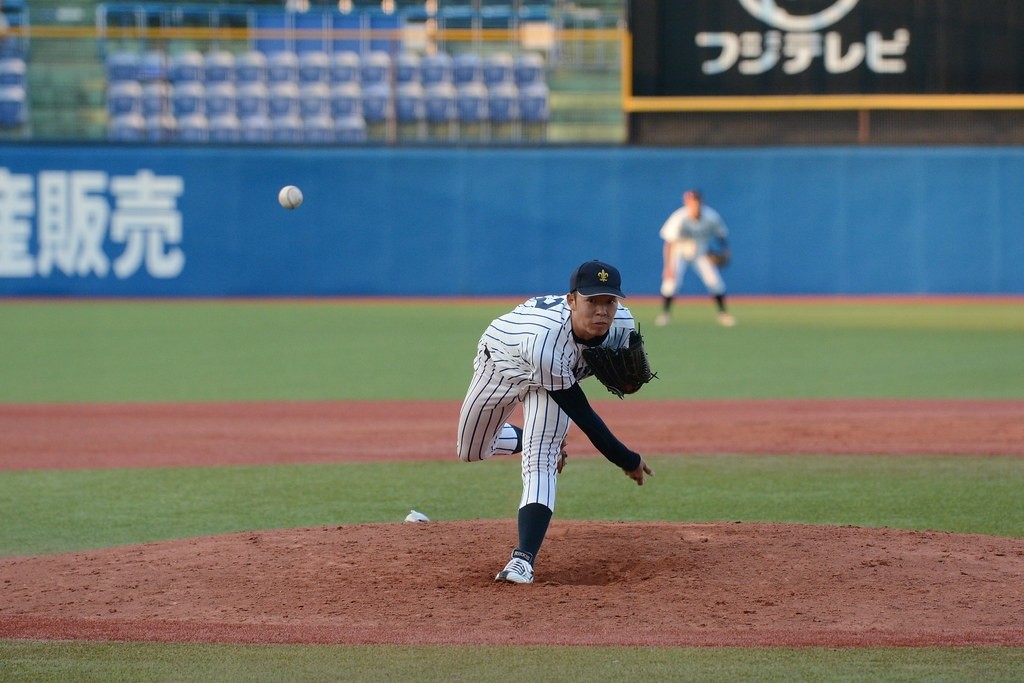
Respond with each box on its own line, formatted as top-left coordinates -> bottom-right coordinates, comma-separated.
570,260 -> 626,299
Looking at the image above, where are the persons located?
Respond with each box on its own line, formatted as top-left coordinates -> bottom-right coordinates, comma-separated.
654,190 -> 735,326
457,260 -> 654,583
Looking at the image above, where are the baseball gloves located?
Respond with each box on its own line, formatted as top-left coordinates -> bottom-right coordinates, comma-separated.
583,331 -> 650,395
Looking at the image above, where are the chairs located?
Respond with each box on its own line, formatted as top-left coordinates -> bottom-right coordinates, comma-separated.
0,0 -> 558,148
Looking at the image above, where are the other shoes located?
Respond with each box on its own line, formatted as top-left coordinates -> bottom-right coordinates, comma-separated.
656,312 -> 673,327
716,311 -> 735,327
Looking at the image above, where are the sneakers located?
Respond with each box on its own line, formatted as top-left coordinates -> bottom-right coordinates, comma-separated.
494,549 -> 535,583
557,436 -> 568,473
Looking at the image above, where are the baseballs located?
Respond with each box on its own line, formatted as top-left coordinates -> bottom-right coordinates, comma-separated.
278,185 -> 303,209
